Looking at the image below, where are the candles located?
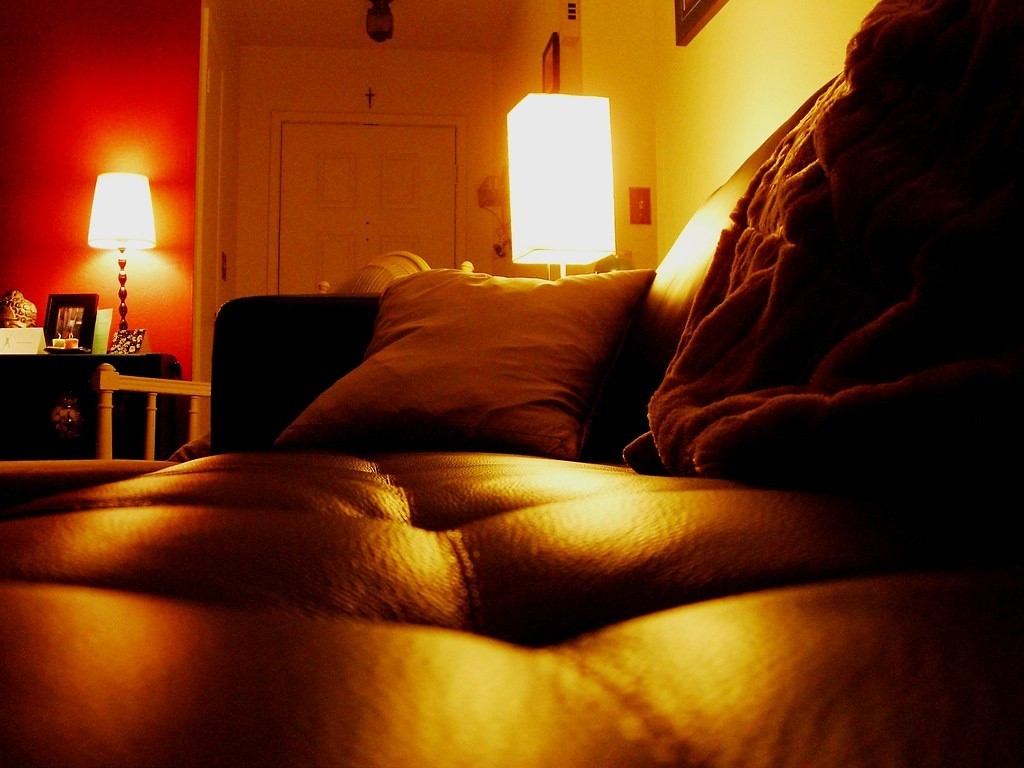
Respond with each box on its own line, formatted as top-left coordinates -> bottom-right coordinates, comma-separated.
66,333 -> 79,349
52,333 -> 66,348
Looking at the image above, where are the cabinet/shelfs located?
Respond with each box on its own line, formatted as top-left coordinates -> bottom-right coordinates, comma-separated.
0,352 -> 185,459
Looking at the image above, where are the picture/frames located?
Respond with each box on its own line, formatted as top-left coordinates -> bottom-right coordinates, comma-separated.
541,30 -> 560,95
42,293 -> 99,351
674,0 -> 729,46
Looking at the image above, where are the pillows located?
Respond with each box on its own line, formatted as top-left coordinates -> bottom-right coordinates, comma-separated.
275,268 -> 654,463
627,0 -> 1024,478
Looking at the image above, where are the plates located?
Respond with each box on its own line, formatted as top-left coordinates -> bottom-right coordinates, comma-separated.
43,347 -> 92,354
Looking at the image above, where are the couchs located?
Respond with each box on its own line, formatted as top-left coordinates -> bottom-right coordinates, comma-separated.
0,74 -> 1024,768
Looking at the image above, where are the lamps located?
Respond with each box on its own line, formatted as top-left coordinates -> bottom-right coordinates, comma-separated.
88,171 -> 156,354
507,93 -> 616,278
